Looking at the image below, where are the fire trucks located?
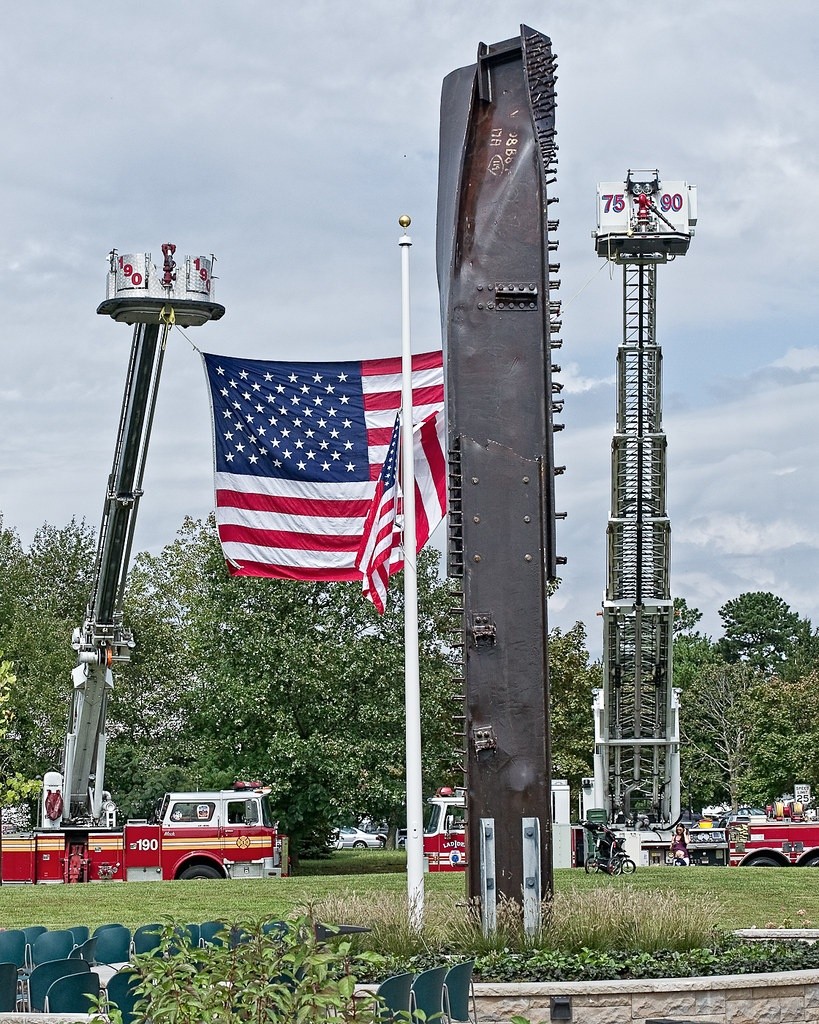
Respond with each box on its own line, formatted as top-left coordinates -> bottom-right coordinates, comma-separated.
0,242 -> 294,881
426,166 -> 726,870
727,797 -> 818,869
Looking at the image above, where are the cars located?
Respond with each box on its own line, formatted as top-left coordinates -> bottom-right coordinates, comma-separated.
328,823 -> 384,849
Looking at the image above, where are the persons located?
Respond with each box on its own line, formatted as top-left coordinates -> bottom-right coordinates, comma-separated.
673,850 -> 687,866
670,823 -> 691,866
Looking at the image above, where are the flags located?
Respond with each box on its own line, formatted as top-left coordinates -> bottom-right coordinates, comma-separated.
199,349 -> 446,617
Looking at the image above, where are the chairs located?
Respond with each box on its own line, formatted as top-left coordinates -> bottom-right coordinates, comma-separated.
0,918 -> 478,1024
